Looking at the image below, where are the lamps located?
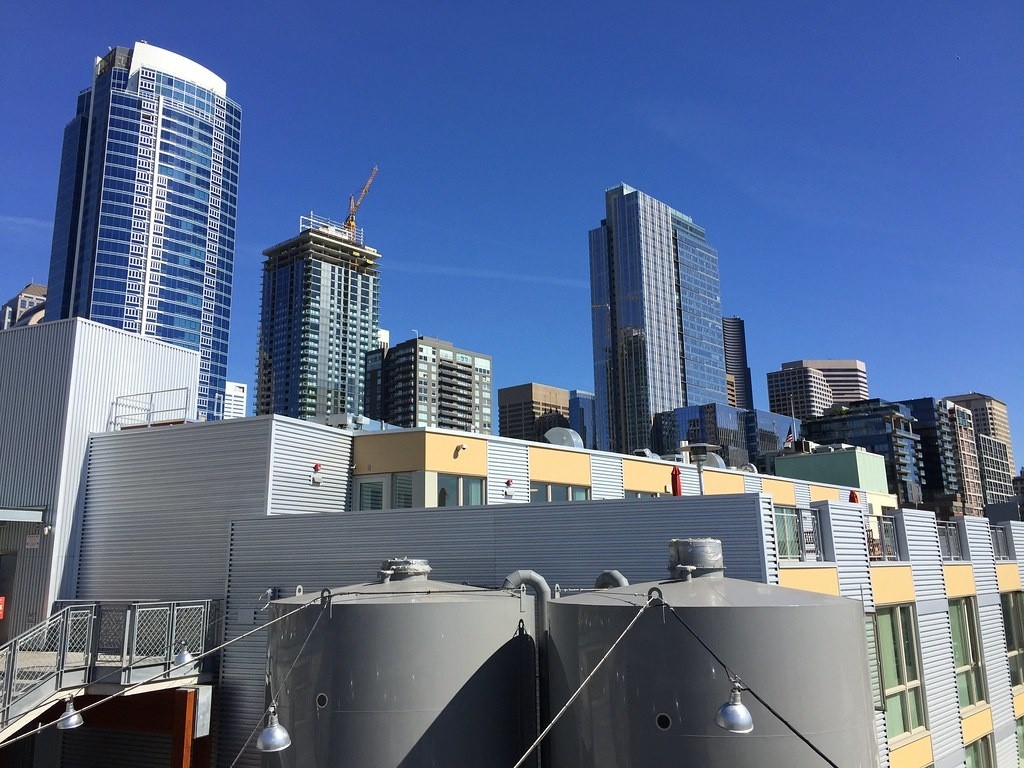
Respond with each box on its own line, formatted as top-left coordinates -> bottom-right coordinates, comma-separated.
714,680 -> 755,733
256,707 -> 291,752
56,697 -> 84,730
174,641 -> 194,667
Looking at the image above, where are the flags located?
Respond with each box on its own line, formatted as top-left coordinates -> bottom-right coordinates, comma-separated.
786,426 -> 793,442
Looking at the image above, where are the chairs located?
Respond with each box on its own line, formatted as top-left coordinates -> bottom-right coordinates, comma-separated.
866,528 -> 893,562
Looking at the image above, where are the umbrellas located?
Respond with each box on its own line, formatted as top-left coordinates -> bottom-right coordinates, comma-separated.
849,491 -> 858,503
671,467 -> 681,496
